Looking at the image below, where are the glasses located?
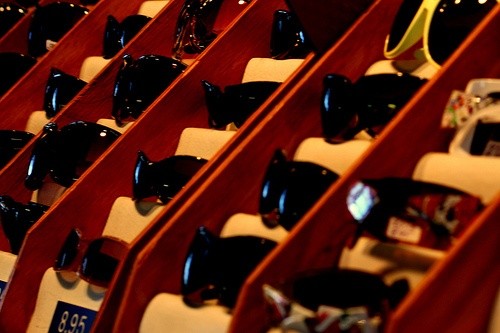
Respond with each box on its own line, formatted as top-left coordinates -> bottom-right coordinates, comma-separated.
1,0 -> 499,333
